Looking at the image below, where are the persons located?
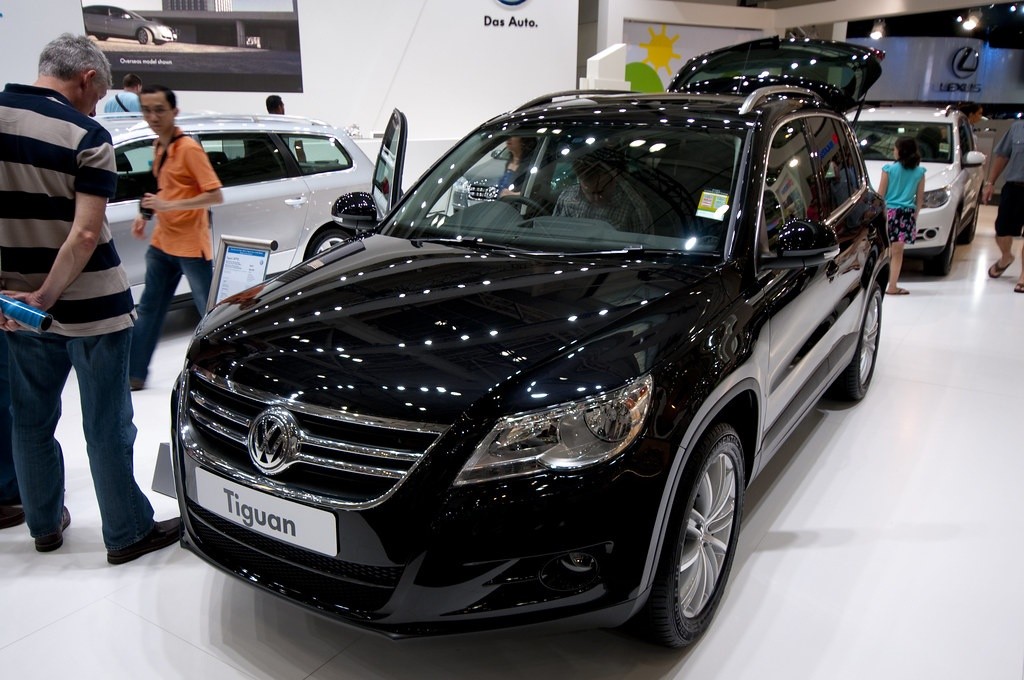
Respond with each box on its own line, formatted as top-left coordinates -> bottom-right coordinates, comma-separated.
975,107 -> 982,122
496,135 -> 538,201
0,33 -> 186,565
130,85 -> 225,391
877,136 -> 927,295
983,120 -> 1024,296
266,95 -> 289,147
552,132 -> 656,236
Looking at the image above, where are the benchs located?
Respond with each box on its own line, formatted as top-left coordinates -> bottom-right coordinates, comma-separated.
206,152 -> 281,185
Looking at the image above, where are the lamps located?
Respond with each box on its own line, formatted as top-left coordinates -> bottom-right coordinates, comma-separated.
962,7 -> 983,31
869,18 -> 886,40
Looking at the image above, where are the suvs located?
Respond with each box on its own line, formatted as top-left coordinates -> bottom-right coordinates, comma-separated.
843,103 -> 988,276
169,31 -> 893,650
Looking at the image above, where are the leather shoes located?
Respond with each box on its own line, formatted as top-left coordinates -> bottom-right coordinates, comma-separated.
35,506 -> 71,552
107,517 -> 185,565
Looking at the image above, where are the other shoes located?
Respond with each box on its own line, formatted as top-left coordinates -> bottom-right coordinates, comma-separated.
129,378 -> 144,389
0,506 -> 25,529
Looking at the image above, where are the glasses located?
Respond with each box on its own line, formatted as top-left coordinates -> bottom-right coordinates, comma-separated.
141,107 -> 170,116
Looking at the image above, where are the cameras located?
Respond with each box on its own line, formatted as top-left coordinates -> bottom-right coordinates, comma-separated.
140,199 -> 154,220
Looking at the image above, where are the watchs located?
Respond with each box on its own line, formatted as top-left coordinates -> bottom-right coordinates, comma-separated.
984,181 -> 994,186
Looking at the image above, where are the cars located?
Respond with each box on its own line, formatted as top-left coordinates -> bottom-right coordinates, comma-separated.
83,4 -> 179,46
450,142 -> 511,212
90,110 -> 446,315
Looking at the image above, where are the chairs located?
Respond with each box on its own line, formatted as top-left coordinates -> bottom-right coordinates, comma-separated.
917,127 -> 944,157
112,152 -> 139,200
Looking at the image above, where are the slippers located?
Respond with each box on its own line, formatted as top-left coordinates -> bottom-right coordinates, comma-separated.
885,288 -> 910,294
988,256 -> 1015,278
1014,283 -> 1024,293
